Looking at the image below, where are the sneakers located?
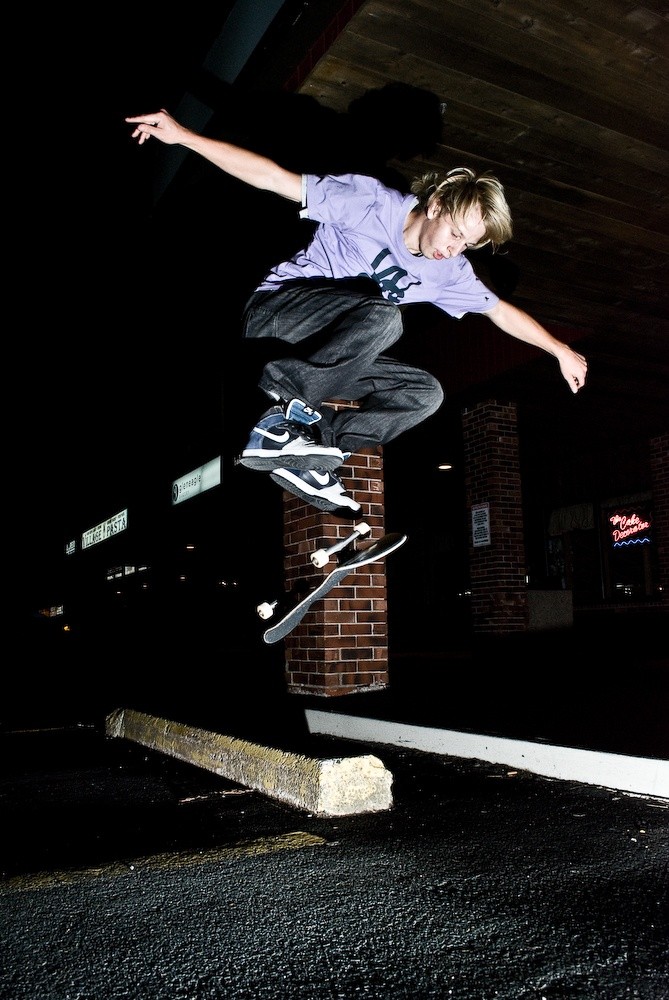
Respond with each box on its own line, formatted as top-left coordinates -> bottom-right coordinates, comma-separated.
269,452 -> 363,520
239,398 -> 344,471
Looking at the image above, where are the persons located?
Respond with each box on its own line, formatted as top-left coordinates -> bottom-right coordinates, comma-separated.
125,109 -> 587,520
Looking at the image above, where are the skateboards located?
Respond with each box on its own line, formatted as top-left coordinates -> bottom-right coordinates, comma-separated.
258,522 -> 410,644
